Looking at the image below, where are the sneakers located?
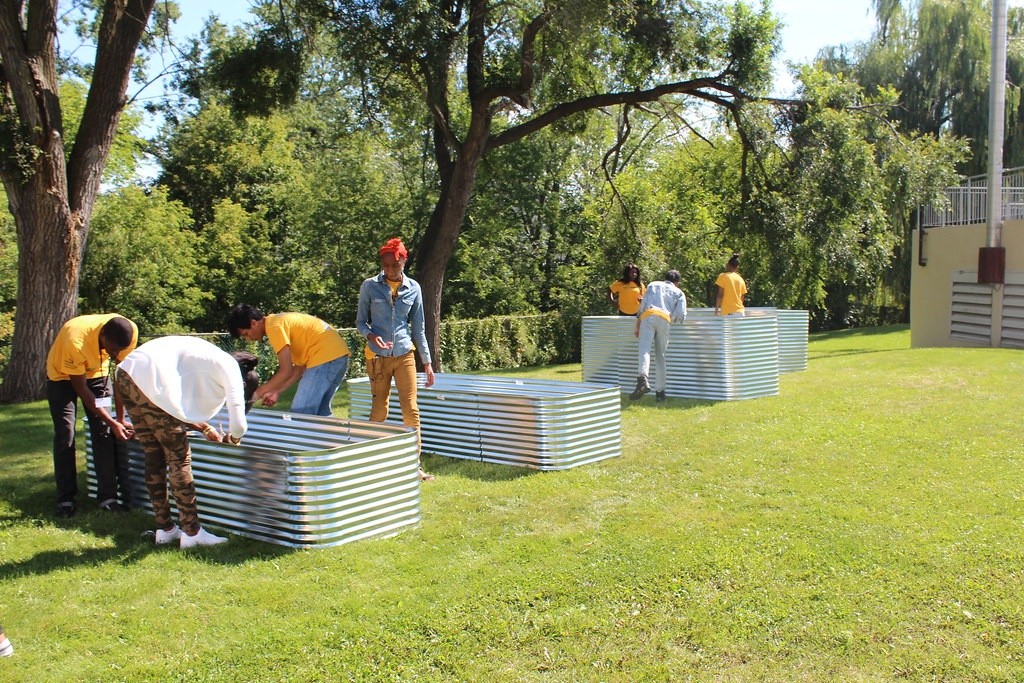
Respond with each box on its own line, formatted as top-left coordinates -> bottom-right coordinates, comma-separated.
156,524 -> 182,544
180,527 -> 228,548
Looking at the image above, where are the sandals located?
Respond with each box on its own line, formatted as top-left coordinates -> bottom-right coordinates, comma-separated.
420,466 -> 434,482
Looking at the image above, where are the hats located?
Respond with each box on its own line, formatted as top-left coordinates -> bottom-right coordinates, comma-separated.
379,238 -> 407,262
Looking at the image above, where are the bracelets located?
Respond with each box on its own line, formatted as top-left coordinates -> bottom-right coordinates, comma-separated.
228,434 -> 242,445
203,426 -> 216,439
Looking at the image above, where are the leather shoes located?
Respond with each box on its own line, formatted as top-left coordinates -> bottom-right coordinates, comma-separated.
102,503 -> 122,512
53,497 -> 77,519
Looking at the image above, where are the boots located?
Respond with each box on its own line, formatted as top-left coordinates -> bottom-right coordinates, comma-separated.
629,377 -> 651,399
656,391 -> 665,401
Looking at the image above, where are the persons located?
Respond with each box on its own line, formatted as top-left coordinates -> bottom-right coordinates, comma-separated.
356,239 -> 435,482
610,264 -> 646,316
115,336 -> 259,547
714,253 -> 747,317
45,313 -> 138,520
630,270 -> 687,402
222,304 -> 349,417
